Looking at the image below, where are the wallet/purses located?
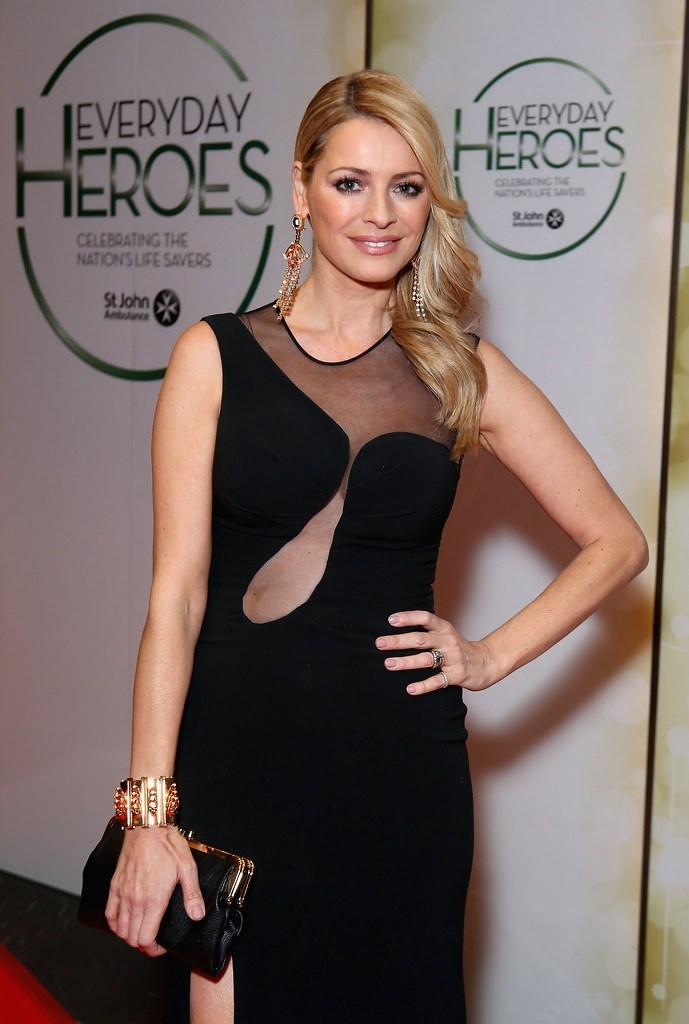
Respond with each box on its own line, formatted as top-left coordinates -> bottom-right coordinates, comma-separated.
79,815 -> 254,979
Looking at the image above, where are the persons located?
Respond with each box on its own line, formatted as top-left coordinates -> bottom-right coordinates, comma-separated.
108,71 -> 649,1024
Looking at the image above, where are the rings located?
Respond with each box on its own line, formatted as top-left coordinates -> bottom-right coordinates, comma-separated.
431,650 -> 445,670
440,672 -> 448,689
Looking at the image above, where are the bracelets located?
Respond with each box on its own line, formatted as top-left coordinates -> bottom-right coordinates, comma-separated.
113,775 -> 180,829
